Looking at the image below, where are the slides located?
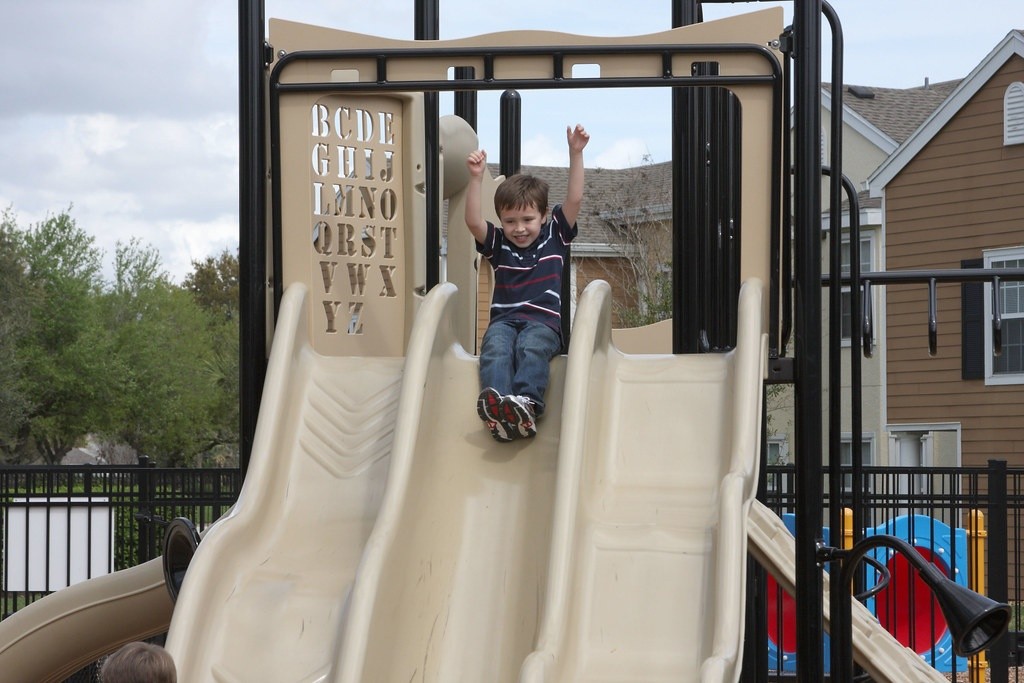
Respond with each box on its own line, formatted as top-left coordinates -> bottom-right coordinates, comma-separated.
164,279 -> 768,683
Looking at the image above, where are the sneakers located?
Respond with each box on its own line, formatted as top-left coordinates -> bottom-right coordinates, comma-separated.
476,387 -> 537,443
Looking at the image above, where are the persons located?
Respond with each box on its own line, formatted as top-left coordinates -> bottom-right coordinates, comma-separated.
101,642 -> 177,683
465,123 -> 590,443
